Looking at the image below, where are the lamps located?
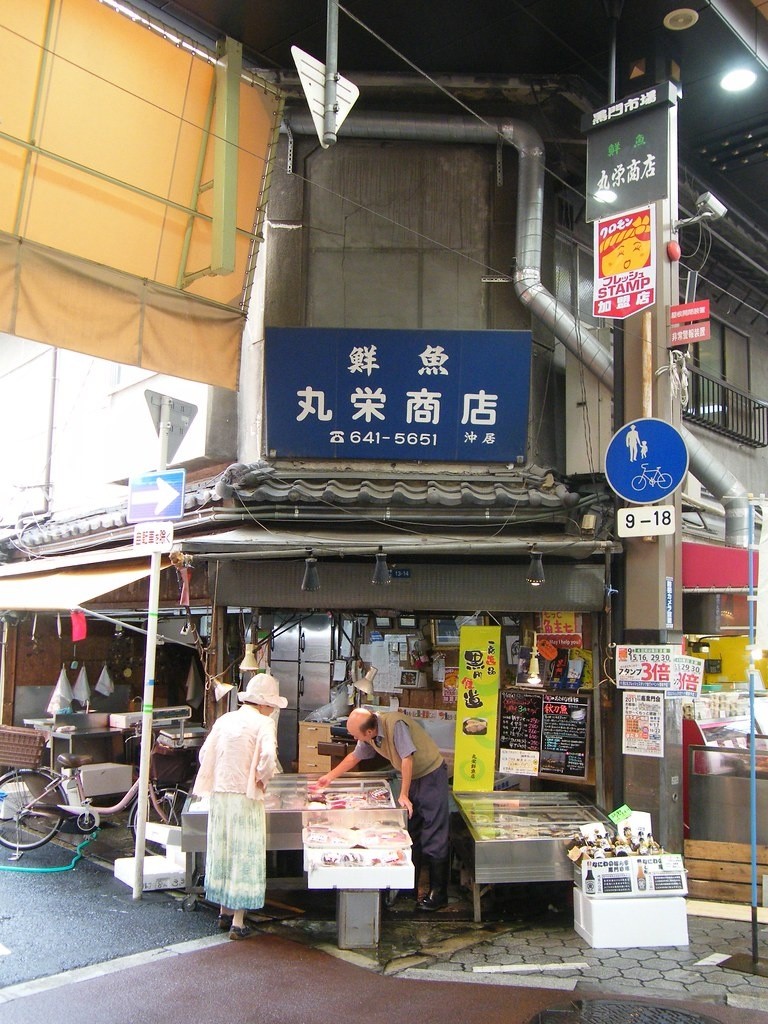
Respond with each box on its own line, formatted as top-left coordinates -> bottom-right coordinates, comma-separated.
239,612 -> 315,670
373,546 -> 392,585
524,629 -> 542,685
301,548 -> 321,591
204,650 -> 235,702
329,611 -> 378,695
526,549 -> 545,586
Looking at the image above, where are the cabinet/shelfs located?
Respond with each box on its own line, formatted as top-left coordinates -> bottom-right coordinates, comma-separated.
298,719 -> 347,774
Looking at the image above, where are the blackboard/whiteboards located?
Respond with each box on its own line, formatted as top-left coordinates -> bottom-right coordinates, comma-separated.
495,689 -> 589,779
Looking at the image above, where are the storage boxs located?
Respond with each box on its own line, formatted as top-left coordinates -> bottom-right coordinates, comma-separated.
307,848 -> 415,890
564,839 -> 689,898
0,782 -> 46,820
60,762 -> 134,797
573,888 -> 689,949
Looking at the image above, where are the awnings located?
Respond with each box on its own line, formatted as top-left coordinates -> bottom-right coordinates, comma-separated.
180,518 -> 618,616
0,550 -> 194,648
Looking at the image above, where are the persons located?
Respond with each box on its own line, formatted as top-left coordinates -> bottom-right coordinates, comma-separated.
193,673 -> 288,940
316,707 -> 452,911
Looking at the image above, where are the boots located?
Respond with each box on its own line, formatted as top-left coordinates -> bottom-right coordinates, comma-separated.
417,857 -> 449,911
400,862 -> 421,898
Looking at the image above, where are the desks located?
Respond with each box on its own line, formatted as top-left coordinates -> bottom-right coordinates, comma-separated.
51,727 -> 130,804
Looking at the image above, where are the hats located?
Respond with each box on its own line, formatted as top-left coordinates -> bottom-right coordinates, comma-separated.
238,673 -> 287,708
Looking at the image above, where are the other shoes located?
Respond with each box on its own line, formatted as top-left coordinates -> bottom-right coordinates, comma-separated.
230,926 -> 252,939
217,914 -> 234,927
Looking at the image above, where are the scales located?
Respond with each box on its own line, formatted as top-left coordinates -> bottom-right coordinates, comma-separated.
152,706 -> 212,750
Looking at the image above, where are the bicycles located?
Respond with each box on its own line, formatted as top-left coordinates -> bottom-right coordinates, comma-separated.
0,719 -> 189,861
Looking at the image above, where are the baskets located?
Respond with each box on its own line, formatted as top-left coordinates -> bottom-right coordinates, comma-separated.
155,752 -> 194,783
0,725 -> 50,768
